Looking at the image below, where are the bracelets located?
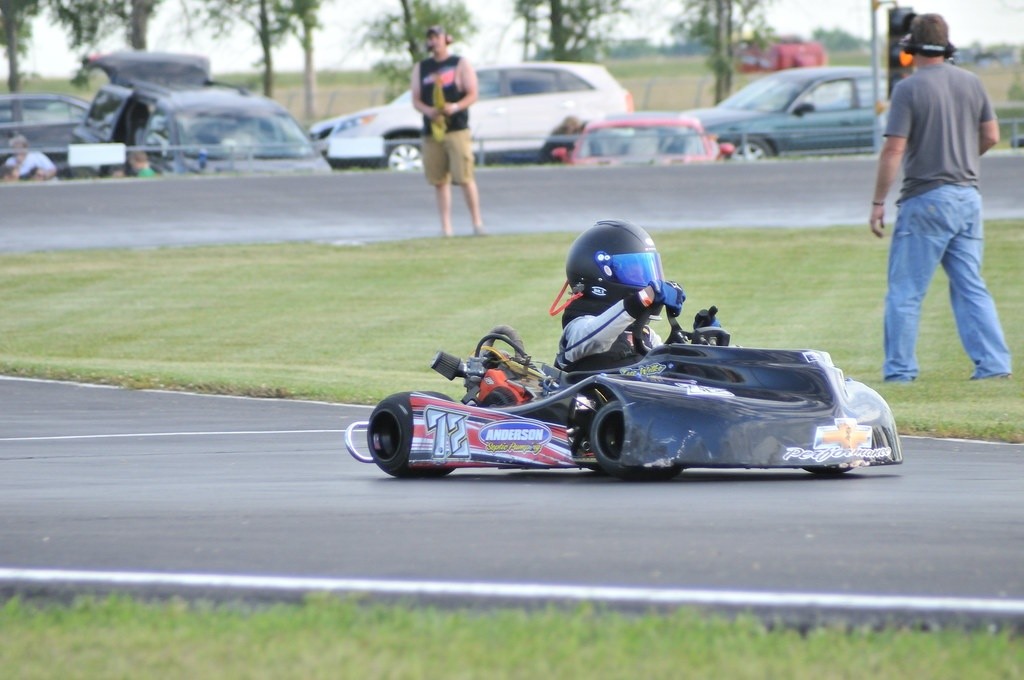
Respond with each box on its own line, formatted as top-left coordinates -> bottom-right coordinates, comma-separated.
452,102 -> 458,115
872,202 -> 884,207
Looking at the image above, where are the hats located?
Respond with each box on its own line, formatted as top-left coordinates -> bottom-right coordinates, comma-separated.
425,25 -> 447,37
899,13 -> 957,57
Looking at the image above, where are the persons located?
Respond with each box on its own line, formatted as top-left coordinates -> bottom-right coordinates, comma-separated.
412,24 -> 488,238
553,219 -> 685,384
536,117 -> 581,165
869,12 -> 1013,381
1,135 -> 163,183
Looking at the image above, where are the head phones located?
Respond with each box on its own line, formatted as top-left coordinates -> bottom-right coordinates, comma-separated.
899,33 -> 958,59
425,32 -> 453,51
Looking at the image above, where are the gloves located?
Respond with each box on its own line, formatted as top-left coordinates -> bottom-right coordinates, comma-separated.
693,306 -> 721,328
649,279 -> 686,317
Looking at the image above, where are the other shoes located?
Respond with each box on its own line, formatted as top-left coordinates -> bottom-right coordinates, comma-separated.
473,226 -> 491,237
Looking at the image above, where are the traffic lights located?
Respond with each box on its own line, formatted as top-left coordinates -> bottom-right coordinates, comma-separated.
885,6 -> 918,101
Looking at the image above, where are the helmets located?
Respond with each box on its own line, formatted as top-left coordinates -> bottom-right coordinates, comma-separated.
565,218 -> 664,315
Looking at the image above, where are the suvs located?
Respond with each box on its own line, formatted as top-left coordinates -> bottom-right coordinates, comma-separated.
311,61 -> 633,172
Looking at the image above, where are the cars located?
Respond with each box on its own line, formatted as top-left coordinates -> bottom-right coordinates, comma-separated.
0,96 -> 97,179
552,114 -> 735,164
81,48 -> 331,174
674,66 -> 891,162
